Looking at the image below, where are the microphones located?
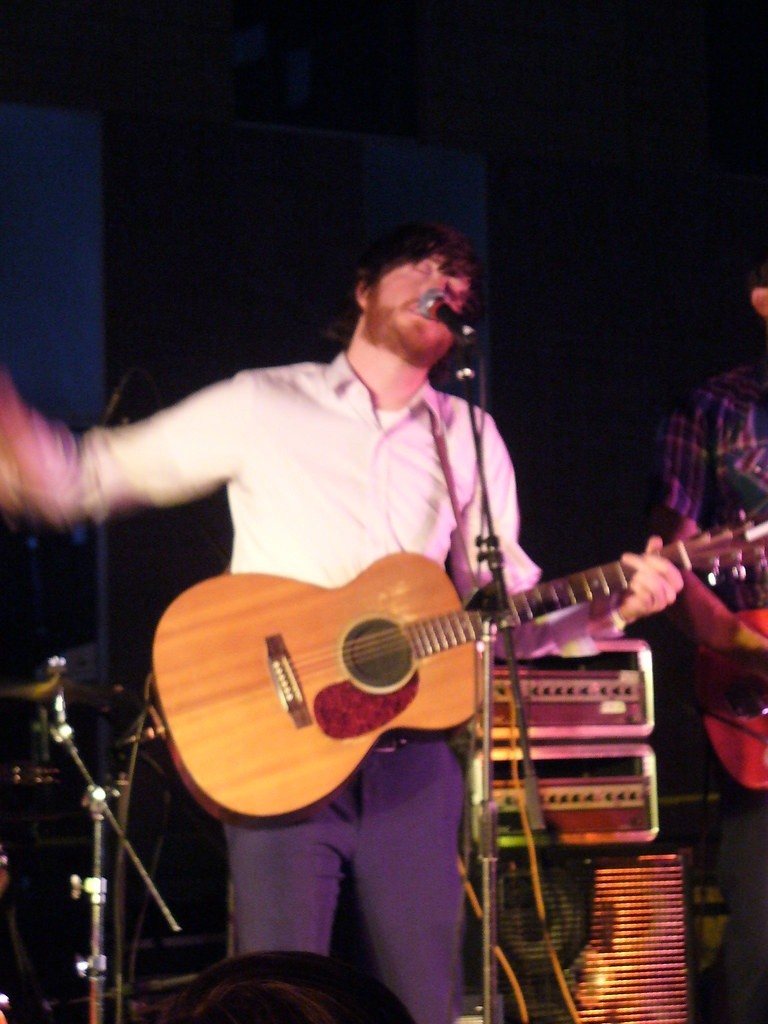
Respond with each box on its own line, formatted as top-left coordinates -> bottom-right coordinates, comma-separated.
422,288 -> 481,345
103,371 -> 135,428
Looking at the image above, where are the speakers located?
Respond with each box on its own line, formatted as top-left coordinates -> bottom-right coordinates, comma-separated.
456,846 -> 697,1024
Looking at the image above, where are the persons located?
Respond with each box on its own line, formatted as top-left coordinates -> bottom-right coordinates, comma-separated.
1,218 -> 685,1023
648,229 -> 768,1024
141,949 -> 416,1024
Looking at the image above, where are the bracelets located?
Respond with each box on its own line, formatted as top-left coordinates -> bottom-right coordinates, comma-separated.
609,590 -> 627,632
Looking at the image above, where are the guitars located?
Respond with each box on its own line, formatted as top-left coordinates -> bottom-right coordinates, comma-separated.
152,510 -> 768,828
699,607 -> 768,790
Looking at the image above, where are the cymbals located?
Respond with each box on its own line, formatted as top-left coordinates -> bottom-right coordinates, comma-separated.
0,762 -> 68,784
0,680 -> 114,710
